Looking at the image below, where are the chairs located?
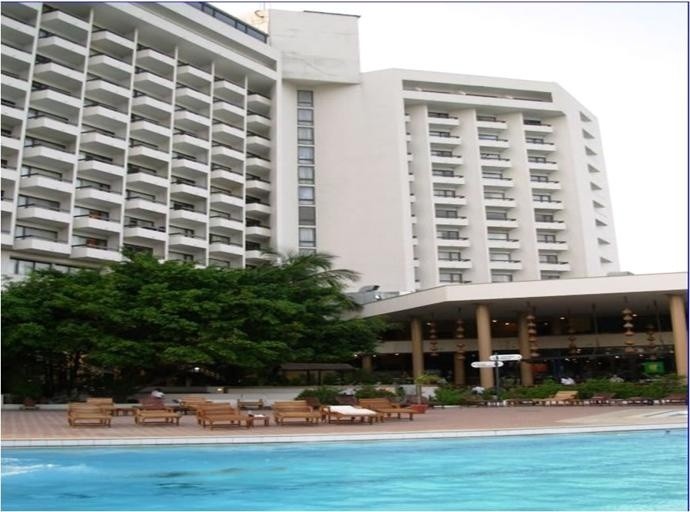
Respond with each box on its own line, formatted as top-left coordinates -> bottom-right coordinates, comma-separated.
461,390 -> 687,407
69,404 -> 112,427
360,398 -> 417,421
133,400 -> 180,425
196,403 -> 242,426
86,397 -> 213,415
273,400 -> 319,426
321,403 -> 376,425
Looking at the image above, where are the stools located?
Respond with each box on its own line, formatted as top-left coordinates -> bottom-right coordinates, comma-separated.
249,414 -> 269,425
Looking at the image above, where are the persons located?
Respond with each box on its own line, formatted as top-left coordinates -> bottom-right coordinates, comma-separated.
561,375 -> 576,386
151,388 -> 164,399
610,375 -> 624,383
471,384 -> 485,394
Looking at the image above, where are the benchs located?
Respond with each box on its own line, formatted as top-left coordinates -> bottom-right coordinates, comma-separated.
238,398 -> 263,409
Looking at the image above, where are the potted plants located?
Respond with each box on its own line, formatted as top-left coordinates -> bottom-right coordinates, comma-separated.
15,385 -> 43,407
